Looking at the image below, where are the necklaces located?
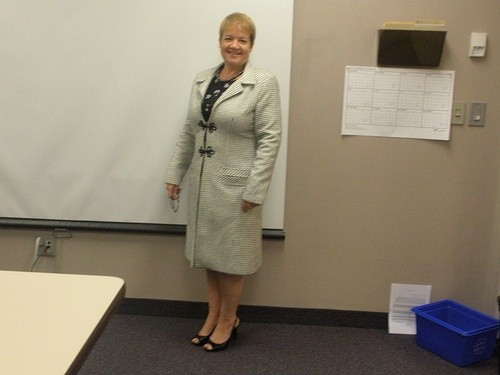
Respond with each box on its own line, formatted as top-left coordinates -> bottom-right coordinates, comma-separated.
215,66 -> 245,82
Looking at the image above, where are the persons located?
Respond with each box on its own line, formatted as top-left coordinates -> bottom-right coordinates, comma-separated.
163,12 -> 281,351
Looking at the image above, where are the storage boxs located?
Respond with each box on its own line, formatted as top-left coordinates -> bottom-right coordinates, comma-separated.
410,299 -> 500,368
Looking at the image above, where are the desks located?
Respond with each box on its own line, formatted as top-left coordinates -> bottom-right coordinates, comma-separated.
0,269 -> 126,375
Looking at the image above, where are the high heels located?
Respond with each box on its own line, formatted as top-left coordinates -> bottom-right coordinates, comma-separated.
190,324 -> 216,347
203,317 -> 240,352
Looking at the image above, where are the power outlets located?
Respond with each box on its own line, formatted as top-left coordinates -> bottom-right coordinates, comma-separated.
36,236 -> 56,257
